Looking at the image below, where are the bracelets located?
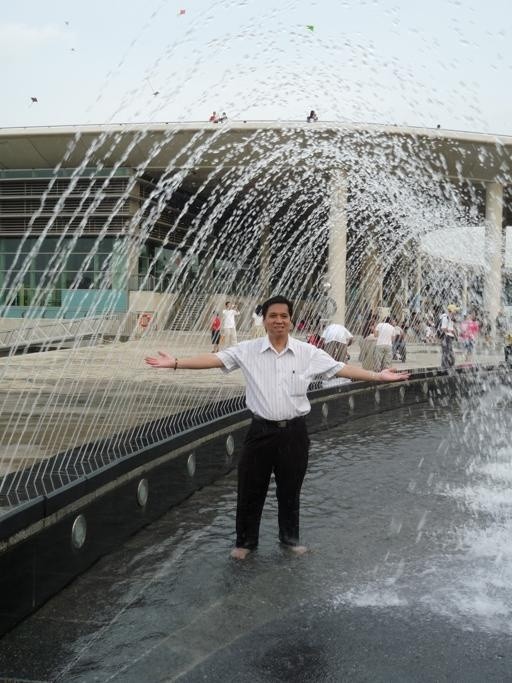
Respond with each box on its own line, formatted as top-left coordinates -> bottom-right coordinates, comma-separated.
174,359 -> 178,369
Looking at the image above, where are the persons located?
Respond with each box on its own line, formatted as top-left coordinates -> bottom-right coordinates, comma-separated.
437,124 -> 440,128
209,112 -> 227,123
210,310 -> 221,352
251,304 -> 265,338
296,304 -> 512,372
145,295 -> 412,561
222,302 -> 240,349
307,111 -> 317,122
